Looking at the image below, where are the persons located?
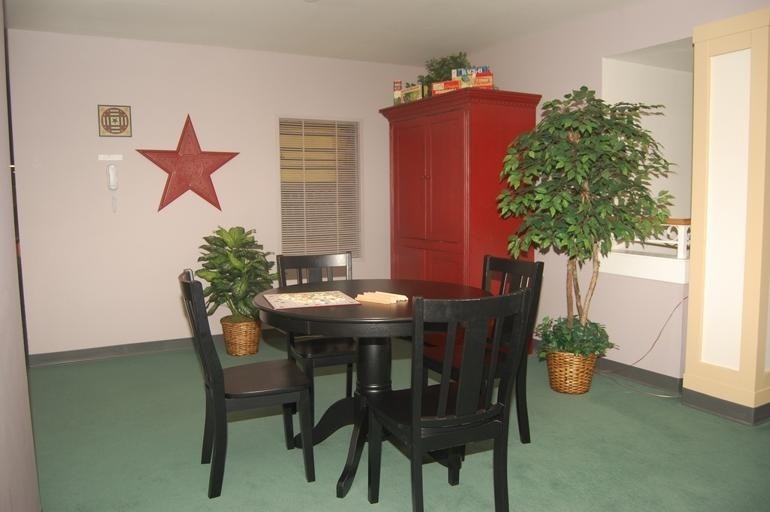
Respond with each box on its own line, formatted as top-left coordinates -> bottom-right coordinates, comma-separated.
667,226 -> 678,242
686,227 -> 691,249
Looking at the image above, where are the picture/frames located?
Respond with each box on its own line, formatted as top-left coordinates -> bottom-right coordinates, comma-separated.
97,102 -> 134,140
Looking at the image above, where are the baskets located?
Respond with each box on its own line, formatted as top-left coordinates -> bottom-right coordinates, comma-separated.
544,349 -> 598,394
219,316 -> 262,357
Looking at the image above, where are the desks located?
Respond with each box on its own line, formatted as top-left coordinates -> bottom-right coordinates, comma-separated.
238,277 -> 508,498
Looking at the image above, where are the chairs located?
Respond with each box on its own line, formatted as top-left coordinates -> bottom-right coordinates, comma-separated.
367,282 -> 535,512
178,268 -> 319,501
418,251 -> 543,449
277,250 -> 371,419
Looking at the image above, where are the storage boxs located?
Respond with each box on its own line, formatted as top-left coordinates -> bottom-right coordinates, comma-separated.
392,65 -> 496,106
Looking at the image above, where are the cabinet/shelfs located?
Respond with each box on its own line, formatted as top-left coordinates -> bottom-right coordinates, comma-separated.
375,86 -> 544,374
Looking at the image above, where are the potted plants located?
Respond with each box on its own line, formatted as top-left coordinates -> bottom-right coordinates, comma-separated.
499,78 -> 674,393
195,222 -> 282,356
